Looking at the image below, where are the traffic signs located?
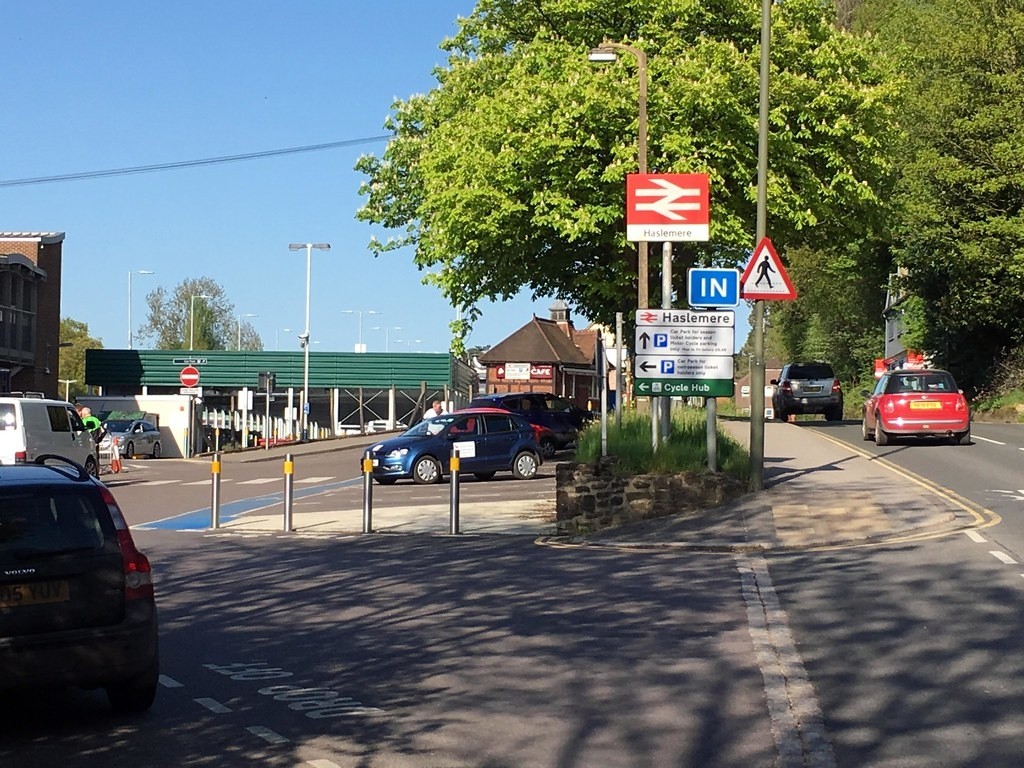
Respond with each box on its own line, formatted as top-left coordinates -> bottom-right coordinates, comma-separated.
634,325 -> 734,397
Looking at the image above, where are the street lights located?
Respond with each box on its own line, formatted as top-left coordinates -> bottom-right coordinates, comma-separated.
57,378 -> 78,402
287,241 -> 332,441
341,310 -> 383,353
274,327 -> 293,351
189,295 -> 214,350
588,40 -> 649,310
128,270 -> 156,349
238,314 -> 260,351
369,325 -> 424,352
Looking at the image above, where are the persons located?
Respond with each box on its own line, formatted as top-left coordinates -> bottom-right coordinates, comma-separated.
424,401 -> 449,435
75,404 -> 107,457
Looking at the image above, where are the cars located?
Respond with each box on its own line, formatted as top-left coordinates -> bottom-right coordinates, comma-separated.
433,407 -> 559,461
859,369 -> 971,446
96,419 -> 163,458
360,410 -> 541,485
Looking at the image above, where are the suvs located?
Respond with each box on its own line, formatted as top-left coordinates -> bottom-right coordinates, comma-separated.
466,391 -> 594,446
768,361 -> 845,422
0,453 -> 160,720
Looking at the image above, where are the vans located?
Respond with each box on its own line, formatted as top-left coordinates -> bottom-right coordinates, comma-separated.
0,396 -> 102,480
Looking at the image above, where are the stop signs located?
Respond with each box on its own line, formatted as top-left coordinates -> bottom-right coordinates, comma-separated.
180,367 -> 200,387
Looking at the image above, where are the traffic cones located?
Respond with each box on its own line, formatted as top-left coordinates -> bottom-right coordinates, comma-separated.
107,436 -> 128,474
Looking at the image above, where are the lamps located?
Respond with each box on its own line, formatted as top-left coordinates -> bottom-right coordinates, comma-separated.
47,343 -> 74,349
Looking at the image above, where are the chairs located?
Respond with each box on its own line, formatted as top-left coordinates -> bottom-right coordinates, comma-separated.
530,398 -> 546,410
509,399 -> 523,409
886,377 -> 904,392
456,418 -> 469,434
925,376 -> 944,391
